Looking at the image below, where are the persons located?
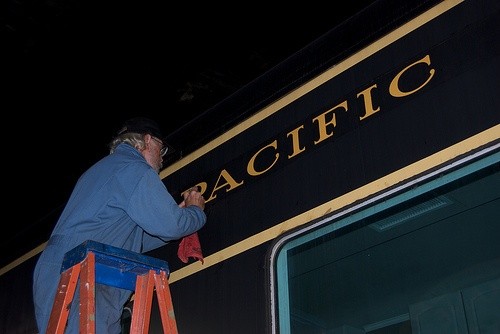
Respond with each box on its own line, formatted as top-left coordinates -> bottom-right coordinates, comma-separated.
33,117 -> 207,334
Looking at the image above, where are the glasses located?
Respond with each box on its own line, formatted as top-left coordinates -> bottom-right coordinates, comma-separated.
151,137 -> 168,156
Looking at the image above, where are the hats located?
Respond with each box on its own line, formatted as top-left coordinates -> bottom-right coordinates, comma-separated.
117,118 -> 176,154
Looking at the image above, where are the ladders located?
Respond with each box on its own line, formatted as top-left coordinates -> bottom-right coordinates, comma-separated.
44,240 -> 179,334
